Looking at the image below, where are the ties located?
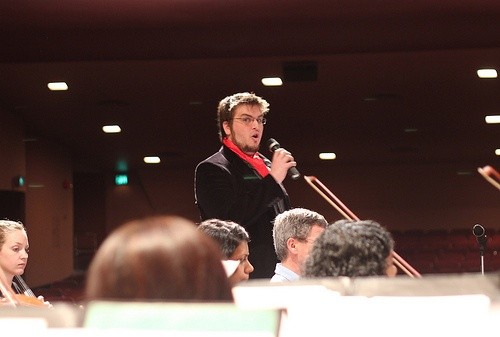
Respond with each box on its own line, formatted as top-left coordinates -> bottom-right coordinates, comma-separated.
222,140 -> 269,178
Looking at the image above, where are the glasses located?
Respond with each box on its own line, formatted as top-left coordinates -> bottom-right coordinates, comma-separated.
224,116 -> 266,125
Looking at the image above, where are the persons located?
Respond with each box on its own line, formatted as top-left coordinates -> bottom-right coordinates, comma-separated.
198,218 -> 254,286
85,216 -> 233,303
194,92 -> 296,279
270,209 -> 328,282
0,219 -> 53,308
305,219 -> 397,277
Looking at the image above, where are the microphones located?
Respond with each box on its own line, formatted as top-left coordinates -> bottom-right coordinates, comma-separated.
473,224 -> 488,246
267,138 -> 300,179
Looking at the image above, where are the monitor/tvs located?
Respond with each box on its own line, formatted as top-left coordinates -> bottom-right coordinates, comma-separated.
0,190 -> 26,224
